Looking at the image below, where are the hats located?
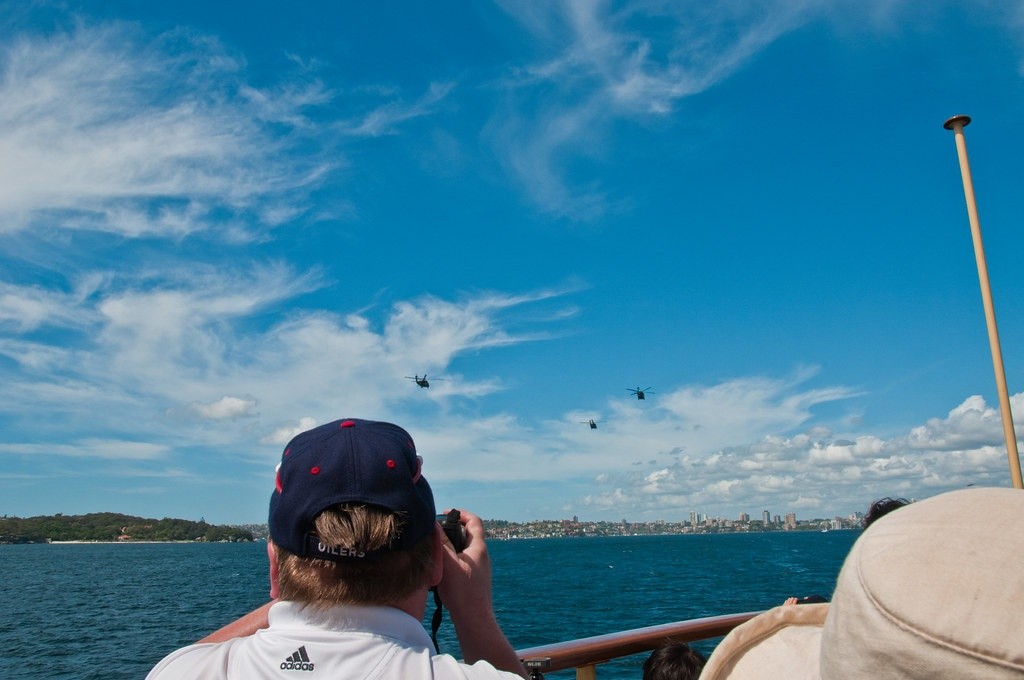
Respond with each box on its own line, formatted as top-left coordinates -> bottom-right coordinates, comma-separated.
268,417 -> 436,565
698,487 -> 1024,680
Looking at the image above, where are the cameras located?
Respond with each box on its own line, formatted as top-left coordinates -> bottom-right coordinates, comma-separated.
435,515 -> 465,554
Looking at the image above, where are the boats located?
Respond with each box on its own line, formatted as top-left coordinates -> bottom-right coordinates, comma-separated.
821,528 -> 830,533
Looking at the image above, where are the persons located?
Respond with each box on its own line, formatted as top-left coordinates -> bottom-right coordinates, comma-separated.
861,497 -> 913,533
783,595 -> 826,607
144,418 -> 530,680
642,641 -> 708,680
698,487 -> 1024,680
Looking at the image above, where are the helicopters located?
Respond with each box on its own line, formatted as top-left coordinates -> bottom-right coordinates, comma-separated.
404,373 -> 444,390
579,418 -> 607,429
626,387 -> 656,401
